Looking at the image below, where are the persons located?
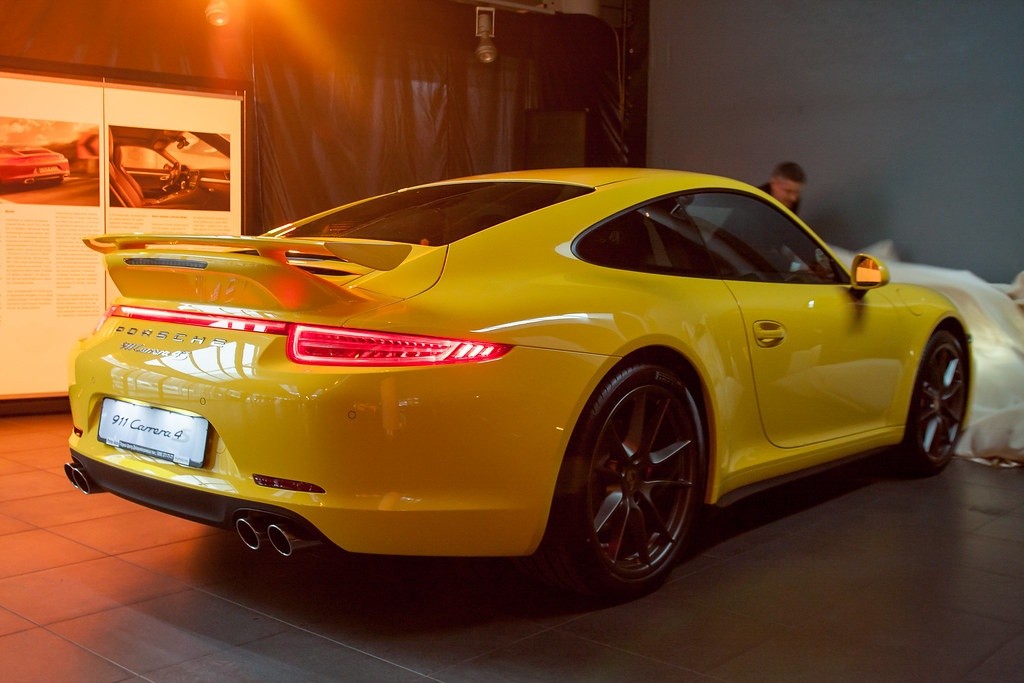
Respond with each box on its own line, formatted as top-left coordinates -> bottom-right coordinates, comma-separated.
706,160 -> 808,275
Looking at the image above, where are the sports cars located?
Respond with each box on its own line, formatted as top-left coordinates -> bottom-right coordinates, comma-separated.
0,145 -> 70,186
64,167 -> 976,612
